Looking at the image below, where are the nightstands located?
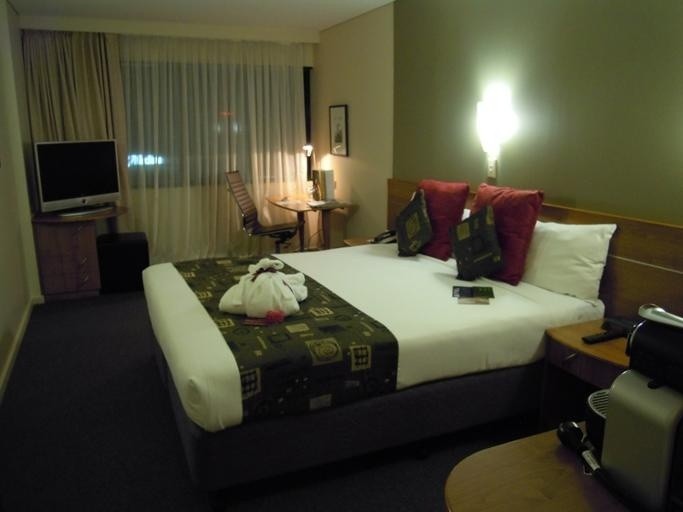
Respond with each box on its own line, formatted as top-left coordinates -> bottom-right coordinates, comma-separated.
538,316 -> 632,434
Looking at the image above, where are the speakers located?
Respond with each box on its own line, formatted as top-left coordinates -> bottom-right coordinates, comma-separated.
97,231 -> 151,295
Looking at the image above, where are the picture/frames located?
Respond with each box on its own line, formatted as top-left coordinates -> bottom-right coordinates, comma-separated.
328,104 -> 348,157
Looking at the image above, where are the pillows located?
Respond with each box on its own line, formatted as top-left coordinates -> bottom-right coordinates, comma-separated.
393,178 -> 619,307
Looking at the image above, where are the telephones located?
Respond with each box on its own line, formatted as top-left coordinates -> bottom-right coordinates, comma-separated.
373,229 -> 398,243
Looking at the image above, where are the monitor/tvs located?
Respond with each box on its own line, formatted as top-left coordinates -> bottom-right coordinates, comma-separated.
33,138 -> 122,216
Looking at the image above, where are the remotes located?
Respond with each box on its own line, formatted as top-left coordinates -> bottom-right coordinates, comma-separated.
582,331 -> 624,344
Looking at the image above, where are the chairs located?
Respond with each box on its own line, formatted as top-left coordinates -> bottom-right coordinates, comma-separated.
224,170 -> 305,253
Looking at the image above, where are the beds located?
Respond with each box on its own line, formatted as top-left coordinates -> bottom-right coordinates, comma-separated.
141,242 -> 606,495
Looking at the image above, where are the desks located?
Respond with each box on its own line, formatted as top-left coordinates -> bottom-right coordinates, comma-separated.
264,193 -> 356,251
